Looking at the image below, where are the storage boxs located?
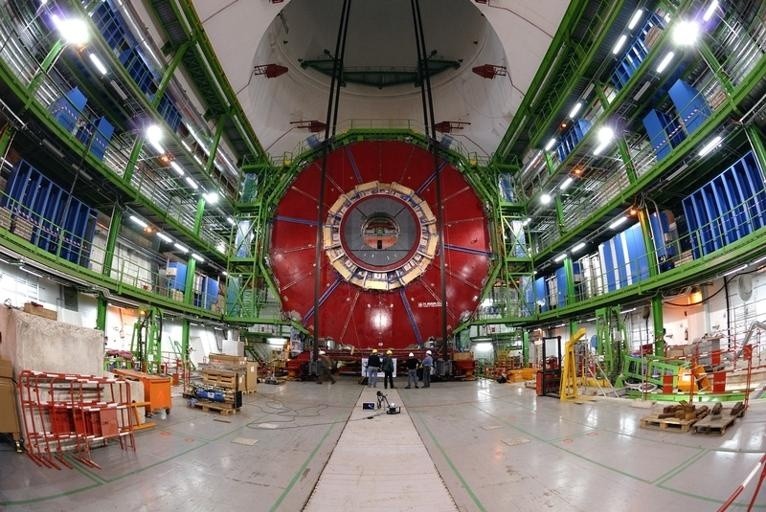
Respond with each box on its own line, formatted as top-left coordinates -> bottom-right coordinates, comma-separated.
23,303 -> 57,320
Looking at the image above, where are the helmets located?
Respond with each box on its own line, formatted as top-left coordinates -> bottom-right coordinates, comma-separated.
426,350 -> 432,355
372,349 -> 378,353
386,350 -> 392,355
409,352 -> 414,357
318,351 -> 326,355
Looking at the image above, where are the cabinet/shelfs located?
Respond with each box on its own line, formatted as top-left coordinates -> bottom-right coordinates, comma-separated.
112,369 -> 172,417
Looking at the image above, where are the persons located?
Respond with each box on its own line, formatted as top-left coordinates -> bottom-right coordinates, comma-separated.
421,350 -> 433,388
316,350 -> 336,384
404,352 -> 421,389
367,349 -> 381,387
382,350 -> 394,389
